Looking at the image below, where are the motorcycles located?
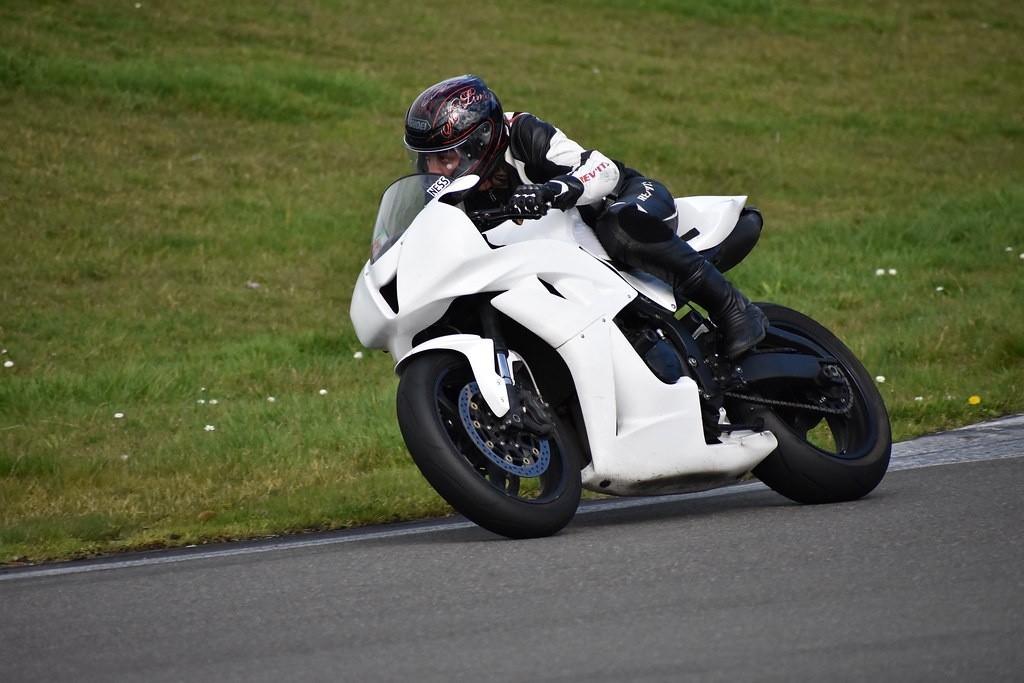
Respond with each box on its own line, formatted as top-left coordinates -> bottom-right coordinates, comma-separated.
349,173 -> 892,539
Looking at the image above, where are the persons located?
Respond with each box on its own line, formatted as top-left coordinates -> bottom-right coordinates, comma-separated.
403,73 -> 769,360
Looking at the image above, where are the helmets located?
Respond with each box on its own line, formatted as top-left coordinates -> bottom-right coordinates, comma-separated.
403,75 -> 510,206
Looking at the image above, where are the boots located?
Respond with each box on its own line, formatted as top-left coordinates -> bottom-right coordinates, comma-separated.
674,260 -> 770,358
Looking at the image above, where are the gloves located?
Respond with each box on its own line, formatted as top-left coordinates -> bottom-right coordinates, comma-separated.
508,185 -> 553,225
